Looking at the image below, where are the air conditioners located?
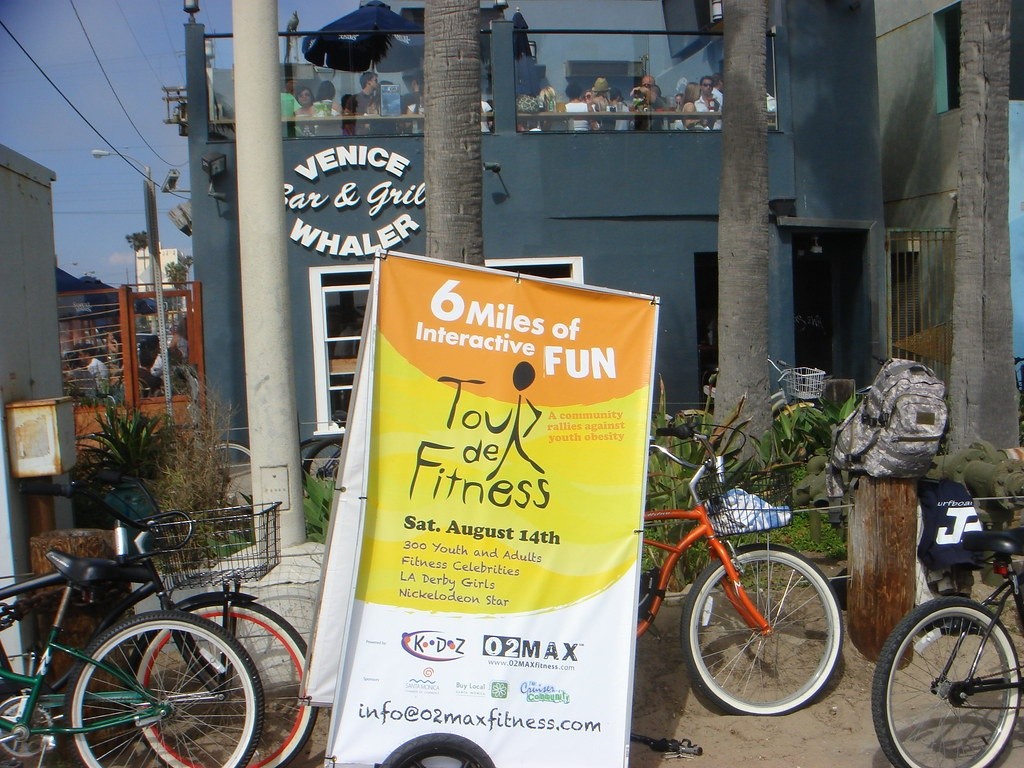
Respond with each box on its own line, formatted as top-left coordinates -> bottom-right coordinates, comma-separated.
177,103 -> 187,121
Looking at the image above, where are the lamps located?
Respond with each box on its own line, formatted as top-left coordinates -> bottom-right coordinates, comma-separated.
809,236 -> 822,253
483,161 -> 510,198
200,152 -> 227,200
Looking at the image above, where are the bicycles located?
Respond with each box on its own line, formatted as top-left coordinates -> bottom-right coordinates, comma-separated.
665,355 -> 830,428
76,423 -> 252,507
871,523 -> 1024,768
0,470 -> 320,768
299,435 -> 344,484
635,416 -> 843,716
0,549 -> 265,768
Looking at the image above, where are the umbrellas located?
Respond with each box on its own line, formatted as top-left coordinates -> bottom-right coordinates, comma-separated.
58,274 -> 159,342
510,6 -> 542,97
302,0 -> 426,75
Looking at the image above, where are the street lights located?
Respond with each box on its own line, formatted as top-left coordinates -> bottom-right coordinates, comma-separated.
92,149 -> 174,428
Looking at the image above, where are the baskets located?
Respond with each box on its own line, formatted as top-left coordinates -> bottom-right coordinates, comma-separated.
695,470 -> 796,538
783,367 -> 825,399
148,501 -> 283,590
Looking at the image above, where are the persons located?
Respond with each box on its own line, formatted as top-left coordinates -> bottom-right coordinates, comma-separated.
514,73 -> 776,133
481,100 -> 494,133
280,68 -> 426,136
61,317 -> 191,405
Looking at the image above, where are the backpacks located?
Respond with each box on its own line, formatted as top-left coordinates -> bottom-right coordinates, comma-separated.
825,357 -> 947,523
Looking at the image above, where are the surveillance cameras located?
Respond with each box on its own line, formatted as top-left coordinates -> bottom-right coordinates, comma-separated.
161,168 -> 180,193
